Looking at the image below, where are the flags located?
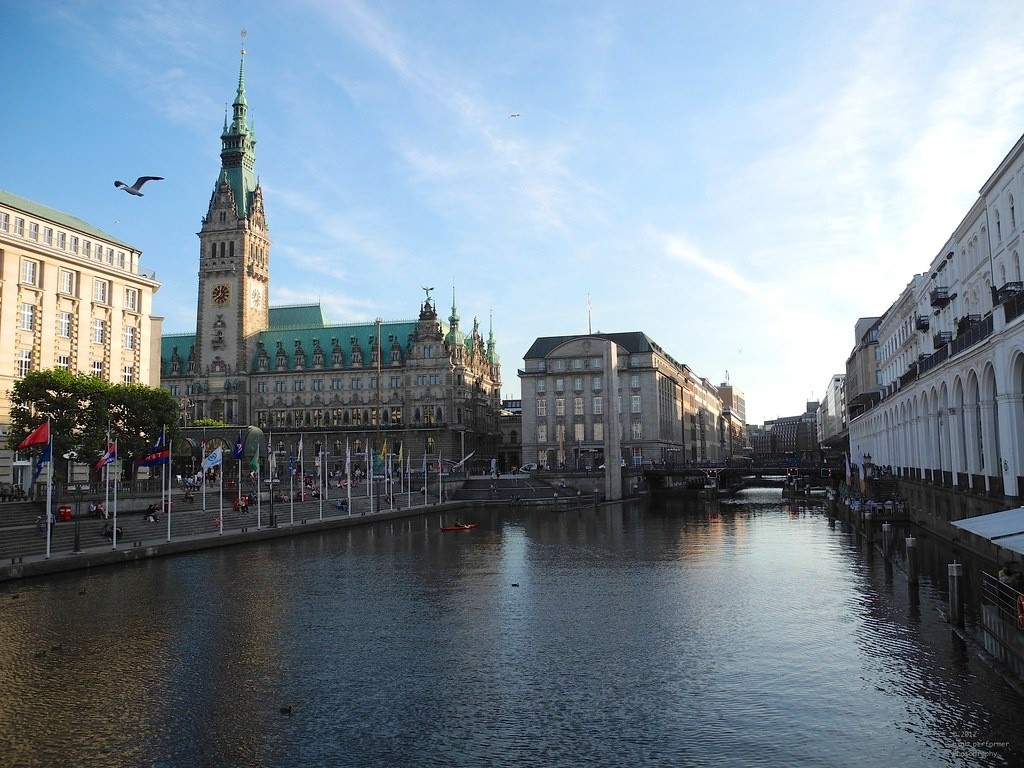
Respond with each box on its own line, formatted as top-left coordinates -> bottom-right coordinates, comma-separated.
233,433 -> 243,460
95,440 -> 116,472
31,446 -> 51,480
856,446 -> 866,494
17,421 -> 50,450
267,431 -> 444,476
845,452 -> 853,487
138,444 -> 171,468
146,430 -> 164,454
200,445 -> 222,472
98,430 -> 111,452
199,433 -> 206,448
250,450 -> 260,472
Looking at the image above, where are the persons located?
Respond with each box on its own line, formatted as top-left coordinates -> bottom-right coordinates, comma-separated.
0,460 -> 462,546
875,466 -> 894,481
994,560 -> 1024,611
844,491 -> 907,516
476,453 -> 845,480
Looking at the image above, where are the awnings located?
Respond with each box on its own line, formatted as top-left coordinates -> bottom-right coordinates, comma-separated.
950,507 -> 1024,560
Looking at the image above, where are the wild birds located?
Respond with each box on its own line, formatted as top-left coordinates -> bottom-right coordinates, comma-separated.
34,650 -> 48,658
509,114 -> 520,118
51,643 -> 63,650
12,593 -> 19,599
280,706 -> 293,714
114,177 -> 164,197
